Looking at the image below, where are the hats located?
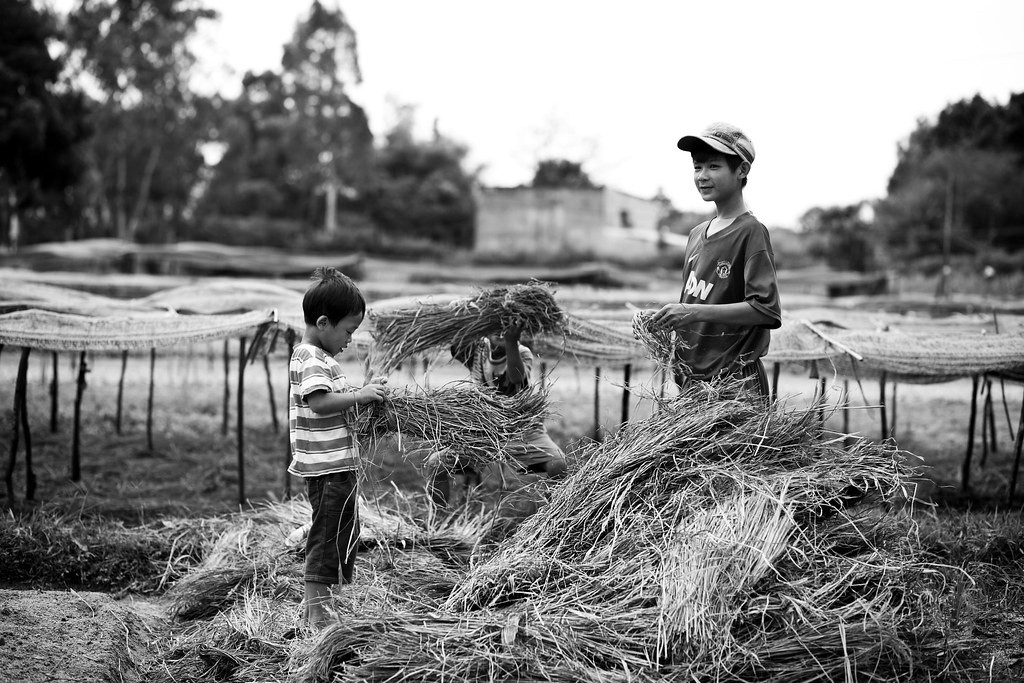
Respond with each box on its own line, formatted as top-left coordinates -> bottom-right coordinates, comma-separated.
677,123 -> 755,166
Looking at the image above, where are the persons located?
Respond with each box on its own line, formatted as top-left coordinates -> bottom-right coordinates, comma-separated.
286,264 -> 391,634
629,124 -> 782,412
